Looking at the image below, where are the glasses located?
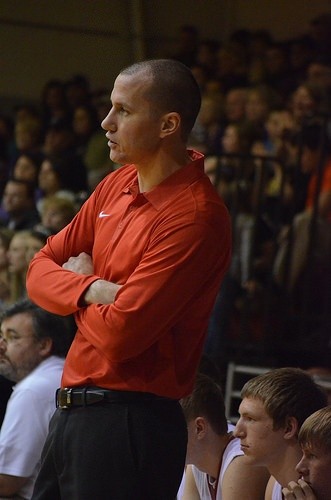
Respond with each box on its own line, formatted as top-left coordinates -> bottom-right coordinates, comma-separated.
0,332 -> 39,347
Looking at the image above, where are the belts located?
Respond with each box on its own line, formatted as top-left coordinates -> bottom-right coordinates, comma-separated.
54,386 -> 163,410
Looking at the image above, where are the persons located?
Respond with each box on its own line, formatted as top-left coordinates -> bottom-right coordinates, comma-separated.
282,407 -> 331,500
234,366 -> 330,500
1,14 -> 330,313
179,372 -> 271,500
26,58 -> 233,500
0,299 -> 71,500
